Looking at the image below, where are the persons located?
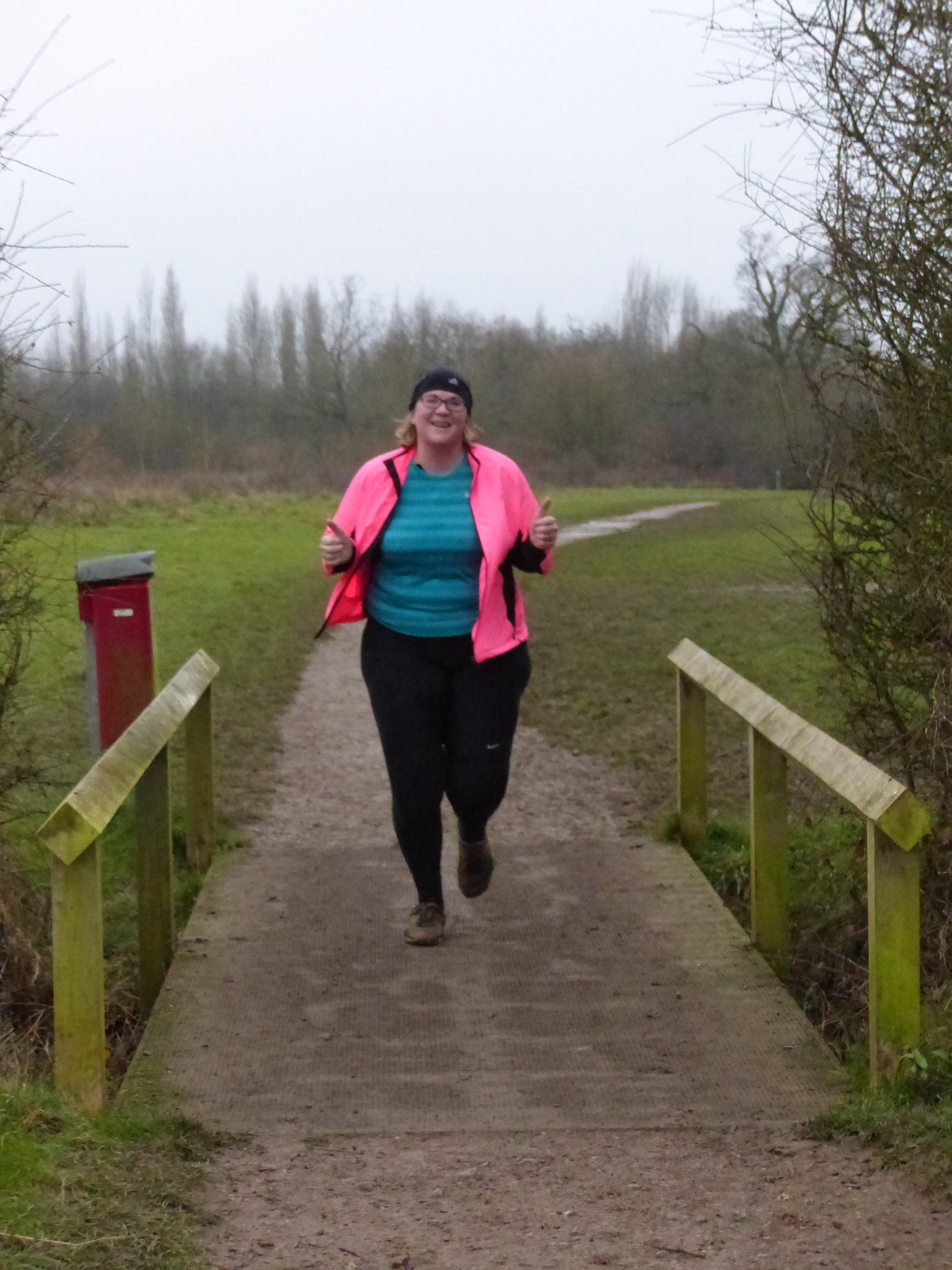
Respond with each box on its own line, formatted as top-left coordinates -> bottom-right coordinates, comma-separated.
310,369 -> 559,944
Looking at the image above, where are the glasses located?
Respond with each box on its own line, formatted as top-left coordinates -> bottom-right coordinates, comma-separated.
418,395 -> 467,412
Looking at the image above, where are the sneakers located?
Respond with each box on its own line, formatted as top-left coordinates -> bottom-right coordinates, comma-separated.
404,902 -> 446,945
457,837 -> 494,899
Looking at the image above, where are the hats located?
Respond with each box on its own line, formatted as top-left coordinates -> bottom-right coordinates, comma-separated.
408,369 -> 473,416
